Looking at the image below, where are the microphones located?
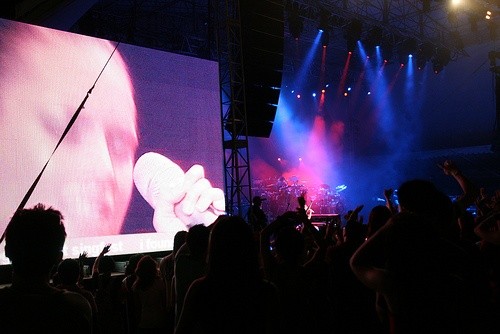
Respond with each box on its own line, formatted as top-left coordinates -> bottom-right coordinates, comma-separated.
133,151 -> 219,229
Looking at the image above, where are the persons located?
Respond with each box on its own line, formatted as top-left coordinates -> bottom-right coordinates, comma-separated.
0,20 -> 225,235
7,170 -> 500,334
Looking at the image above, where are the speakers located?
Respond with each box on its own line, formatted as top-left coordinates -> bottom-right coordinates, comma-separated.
225,10 -> 284,138
